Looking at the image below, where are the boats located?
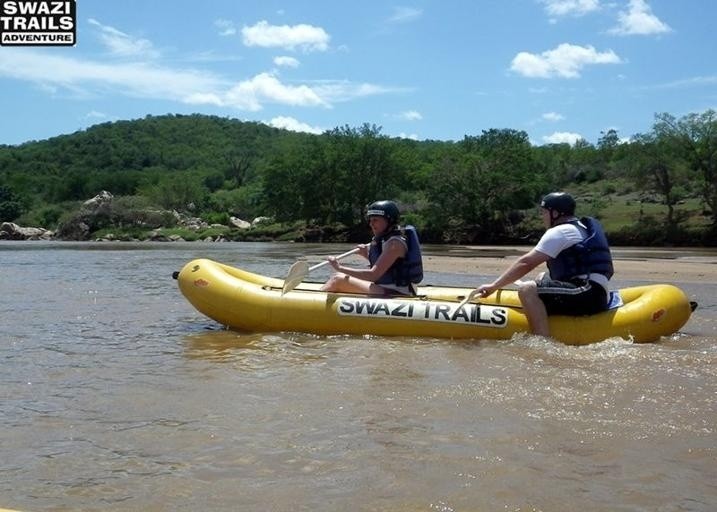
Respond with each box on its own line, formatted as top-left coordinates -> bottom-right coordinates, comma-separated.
169,256 -> 700,345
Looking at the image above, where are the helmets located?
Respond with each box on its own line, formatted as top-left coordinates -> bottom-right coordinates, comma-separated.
366,200 -> 400,226
539,191 -> 576,211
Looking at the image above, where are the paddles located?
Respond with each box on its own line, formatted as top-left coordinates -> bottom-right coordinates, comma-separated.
280,242 -> 375,296
454,279 -> 522,316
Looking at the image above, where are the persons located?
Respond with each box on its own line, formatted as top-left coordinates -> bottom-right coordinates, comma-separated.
474,191 -> 614,340
321,199 -> 424,296
